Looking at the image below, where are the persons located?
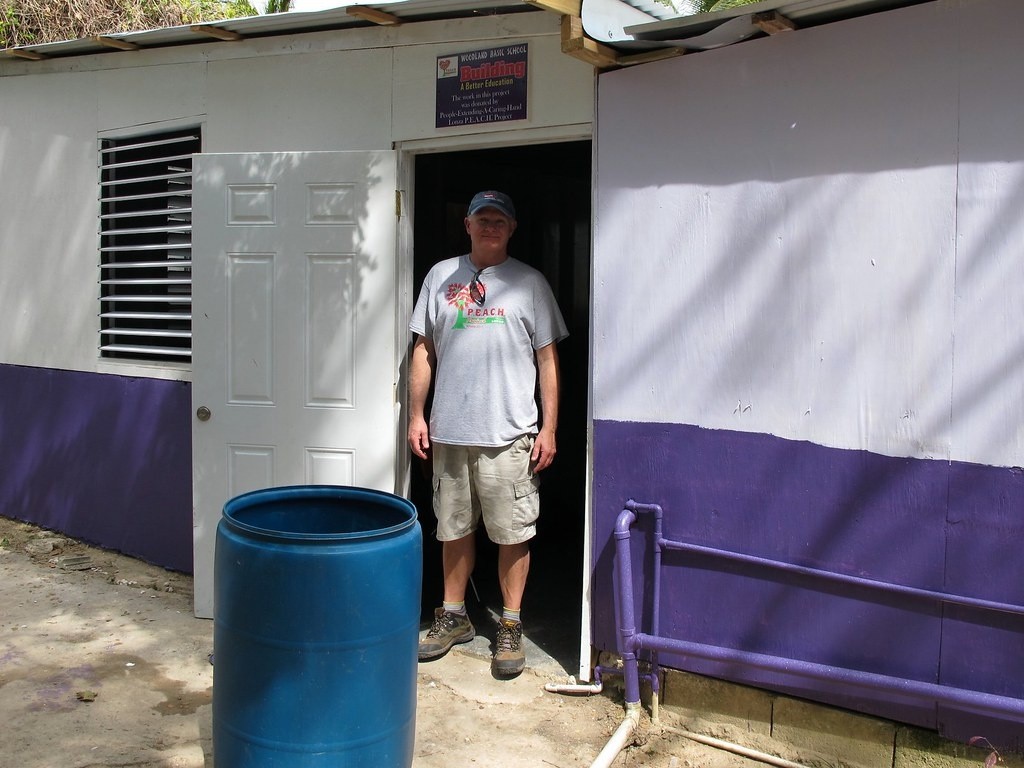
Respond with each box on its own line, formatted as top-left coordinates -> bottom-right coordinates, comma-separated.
409,190 -> 569,677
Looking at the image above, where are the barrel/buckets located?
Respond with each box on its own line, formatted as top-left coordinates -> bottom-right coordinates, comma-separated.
212,485 -> 423,768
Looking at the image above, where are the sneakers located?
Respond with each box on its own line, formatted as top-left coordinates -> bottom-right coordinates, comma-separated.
417,608 -> 475,660
492,618 -> 526,674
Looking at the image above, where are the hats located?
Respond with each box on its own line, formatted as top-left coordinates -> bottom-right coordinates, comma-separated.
467,191 -> 514,218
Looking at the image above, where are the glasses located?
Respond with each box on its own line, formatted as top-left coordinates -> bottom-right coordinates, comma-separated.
470,269 -> 486,304
